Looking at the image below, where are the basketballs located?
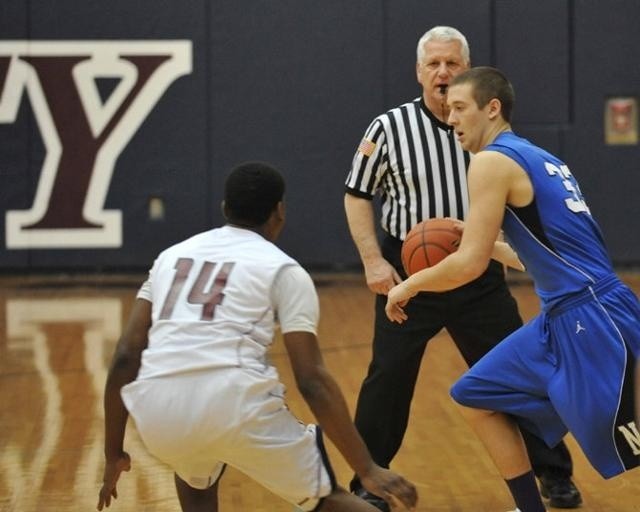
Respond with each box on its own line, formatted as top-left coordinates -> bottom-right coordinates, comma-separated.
401,218 -> 465,277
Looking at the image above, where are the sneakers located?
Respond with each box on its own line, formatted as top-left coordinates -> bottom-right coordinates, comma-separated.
538,472 -> 583,509
347,472 -> 397,512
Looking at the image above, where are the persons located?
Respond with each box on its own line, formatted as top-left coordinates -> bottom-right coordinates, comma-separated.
98,161 -> 419,512
351,24 -> 584,511
383,64 -> 640,511
